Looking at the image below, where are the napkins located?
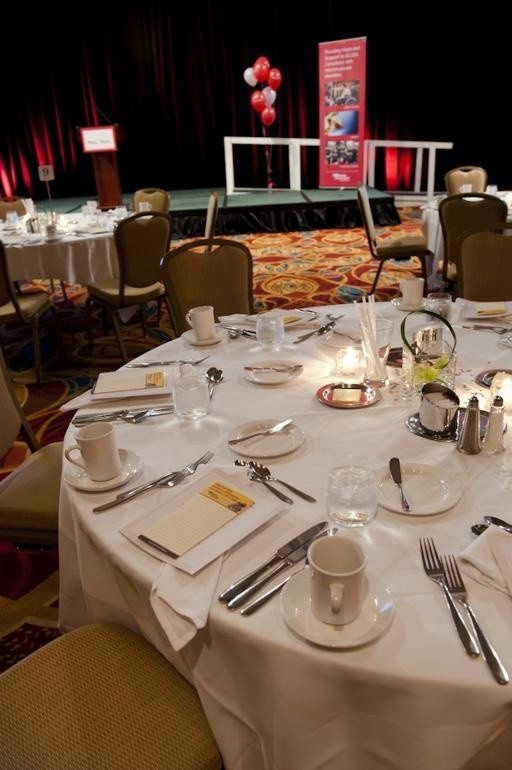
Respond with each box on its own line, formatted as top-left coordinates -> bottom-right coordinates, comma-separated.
59,389 -> 127,412
457,524 -> 512,597
150,549 -> 224,652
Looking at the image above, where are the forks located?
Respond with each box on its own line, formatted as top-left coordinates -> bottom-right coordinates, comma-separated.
417,537 -> 480,659
93,449 -> 213,515
125,355 -> 211,369
439,553 -> 509,687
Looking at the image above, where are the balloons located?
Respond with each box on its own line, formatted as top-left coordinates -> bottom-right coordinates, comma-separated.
242,54 -> 282,127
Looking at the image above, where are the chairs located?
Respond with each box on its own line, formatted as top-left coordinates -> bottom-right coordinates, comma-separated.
0,353 -> 64,549
0,621 -> 224,770
357,184 -> 432,298
1,189 -> 255,391
439,166 -> 512,302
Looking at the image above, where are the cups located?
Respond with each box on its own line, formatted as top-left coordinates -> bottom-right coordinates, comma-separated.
360,319 -> 394,387
186,306 -> 216,341
329,466 -> 382,531
418,382 -> 458,436
172,367 -> 210,420
429,293 -> 453,325
308,538 -> 366,626
255,312 -> 286,349
399,277 -> 423,304
6,200 -> 127,239
66,422 -> 120,482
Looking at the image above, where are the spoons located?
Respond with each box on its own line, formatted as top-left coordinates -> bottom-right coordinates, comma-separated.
206,365 -> 223,398
249,461 -> 316,504
473,513 -> 512,536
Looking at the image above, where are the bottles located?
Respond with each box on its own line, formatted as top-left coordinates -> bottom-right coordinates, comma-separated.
459,398 -> 481,455
483,396 -> 506,454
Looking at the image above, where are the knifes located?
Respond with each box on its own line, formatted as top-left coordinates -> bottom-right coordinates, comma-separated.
228,417 -> 293,448
70,404 -> 175,427
219,521 -> 340,617
388,454 -> 410,511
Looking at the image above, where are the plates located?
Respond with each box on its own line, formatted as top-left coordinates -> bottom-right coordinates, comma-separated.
392,297 -> 425,310
378,462 -> 463,516
63,447 -> 140,492
477,368 -> 511,388
405,406 -> 506,444
241,327 -> 403,411
182,328 -> 225,345
278,569 -> 396,647
229,420 -> 306,459
